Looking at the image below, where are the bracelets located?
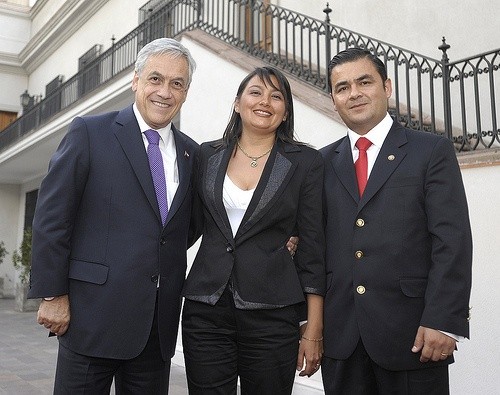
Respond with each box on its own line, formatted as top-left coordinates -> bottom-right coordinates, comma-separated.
302,336 -> 323,342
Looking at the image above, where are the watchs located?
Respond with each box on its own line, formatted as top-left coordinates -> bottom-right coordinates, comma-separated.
41,297 -> 54,302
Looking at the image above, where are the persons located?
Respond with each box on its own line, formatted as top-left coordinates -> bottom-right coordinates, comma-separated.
298,48 -> 473,395
182,65 -> 328,395
25,37 -> 299,395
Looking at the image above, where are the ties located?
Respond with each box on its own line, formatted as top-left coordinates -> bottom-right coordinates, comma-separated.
143,129 -> 168,229
354,137 -> 373,200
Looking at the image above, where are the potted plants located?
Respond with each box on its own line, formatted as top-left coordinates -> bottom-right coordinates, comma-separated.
13,227 -> 43,312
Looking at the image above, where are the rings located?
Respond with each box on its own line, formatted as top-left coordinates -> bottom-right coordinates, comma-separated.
314,367 -> 317,369
441,353 -> 448,358
317,363 -> 320,366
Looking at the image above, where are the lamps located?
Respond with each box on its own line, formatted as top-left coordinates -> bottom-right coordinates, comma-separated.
19,90 -> 31,106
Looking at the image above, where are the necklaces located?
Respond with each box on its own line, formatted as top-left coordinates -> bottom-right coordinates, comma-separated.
238,141 -> 273,168
